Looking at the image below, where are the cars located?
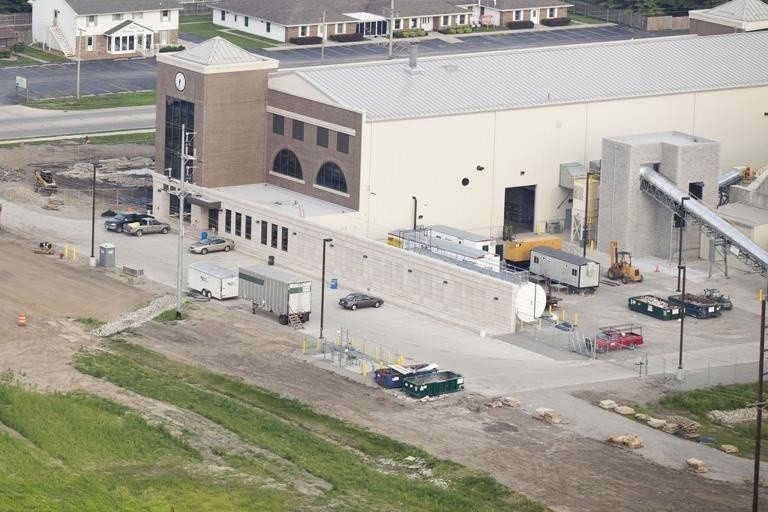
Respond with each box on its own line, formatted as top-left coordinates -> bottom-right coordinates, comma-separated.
188,235 -> 235,256
338,293 -> 383,311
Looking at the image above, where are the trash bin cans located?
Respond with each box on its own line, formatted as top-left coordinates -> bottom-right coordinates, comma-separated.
201,231 -> 208,239
330,278 -> 337,289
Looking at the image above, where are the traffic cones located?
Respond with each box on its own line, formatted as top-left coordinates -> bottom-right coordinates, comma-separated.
17,309 -> 27,327
654,263 -> 659,273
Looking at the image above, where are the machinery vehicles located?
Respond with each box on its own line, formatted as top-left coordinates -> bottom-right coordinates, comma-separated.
604,240 -> 645,284
699,287 -> 731,313
32,169 -> 57,195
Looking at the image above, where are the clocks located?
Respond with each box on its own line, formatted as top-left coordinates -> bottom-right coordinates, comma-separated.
174,72 -> 186,91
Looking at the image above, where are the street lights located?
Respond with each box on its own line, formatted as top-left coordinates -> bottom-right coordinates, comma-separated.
90,164 -> 103,257
676,264 -> 686,370
75,27 -> 87,101
320,237 -> 333,338
411,196 -> 417,230
676,197 -> 691,293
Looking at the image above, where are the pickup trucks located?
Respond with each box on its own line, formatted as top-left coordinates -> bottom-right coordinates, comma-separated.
104,213 -> 171,237
586,323 -> 643,353
